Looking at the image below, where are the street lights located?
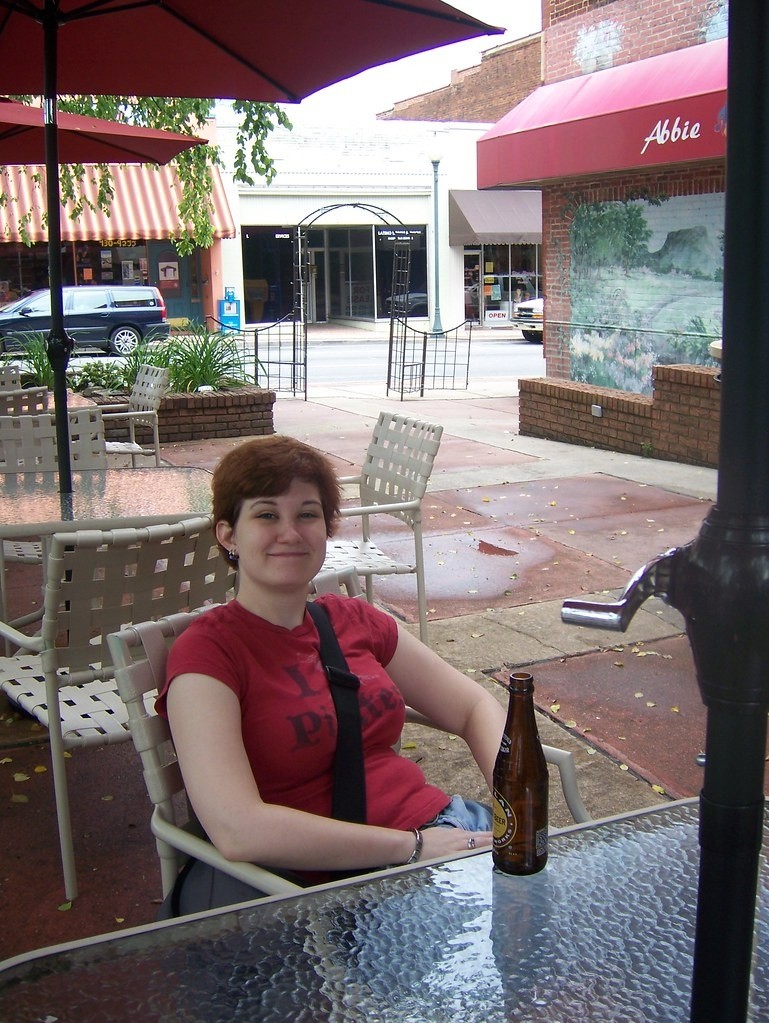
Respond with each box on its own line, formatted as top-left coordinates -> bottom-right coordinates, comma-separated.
427,148 -> 445,339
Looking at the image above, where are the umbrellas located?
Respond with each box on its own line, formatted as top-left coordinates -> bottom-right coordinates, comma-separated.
0,1 -> 506,648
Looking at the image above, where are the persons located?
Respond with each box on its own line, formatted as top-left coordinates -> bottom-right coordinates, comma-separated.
154,436 -> 507,888
514,288 -> 530,304
464,286 -> 476,321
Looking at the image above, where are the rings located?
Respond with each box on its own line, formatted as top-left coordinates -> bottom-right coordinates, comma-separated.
467,836 -> 476,849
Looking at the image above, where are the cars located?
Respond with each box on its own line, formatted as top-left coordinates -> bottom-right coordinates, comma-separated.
512,298 -> 544,343
387,273 -> 542,317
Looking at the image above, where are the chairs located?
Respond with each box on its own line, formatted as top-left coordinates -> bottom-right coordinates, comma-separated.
0,365 -> 107,470
320,409 -> 445,647
76,365 -> 170,467
0,513 -> 241,901
103,567 -> 593,903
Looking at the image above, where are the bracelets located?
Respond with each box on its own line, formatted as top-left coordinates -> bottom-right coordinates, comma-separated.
404,826 -> 423,865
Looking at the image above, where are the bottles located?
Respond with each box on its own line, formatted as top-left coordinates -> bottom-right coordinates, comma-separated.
491,668 -> 551,878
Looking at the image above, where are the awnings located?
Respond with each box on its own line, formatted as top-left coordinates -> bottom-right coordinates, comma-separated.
449,190 -> 543,246
0,163 -> 236,243
477,37 -> 730,194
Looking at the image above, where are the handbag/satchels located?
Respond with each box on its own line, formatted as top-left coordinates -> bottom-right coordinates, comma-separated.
153,837 -> 300,924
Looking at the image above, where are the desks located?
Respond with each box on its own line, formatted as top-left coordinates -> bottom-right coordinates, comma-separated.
0,797 -> 769,1023
0,469 -> 218,537
7,389 -> 97,415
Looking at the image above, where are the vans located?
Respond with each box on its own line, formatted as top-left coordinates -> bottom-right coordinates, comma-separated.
0,285 -> 171,360
